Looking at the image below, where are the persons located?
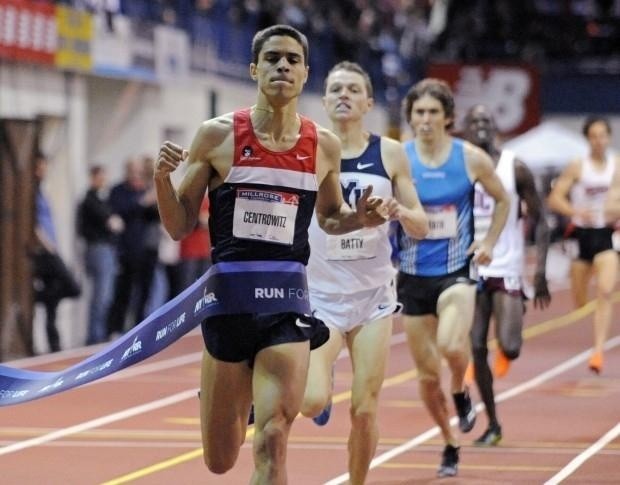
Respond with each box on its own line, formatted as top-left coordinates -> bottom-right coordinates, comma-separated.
463,105 -> 550,445
547,115 -> 620,376
34,150 -> 211,352
390,76 -> 509,478
301,60 -> 431,484
154,24 -> 390,485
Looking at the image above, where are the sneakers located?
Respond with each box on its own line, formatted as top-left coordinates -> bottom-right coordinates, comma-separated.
312,403 -> 333,425
493,345 -> 512,380
436,444 -> 460,478
452,388 -> 502,446
588,352 -> 604,374
